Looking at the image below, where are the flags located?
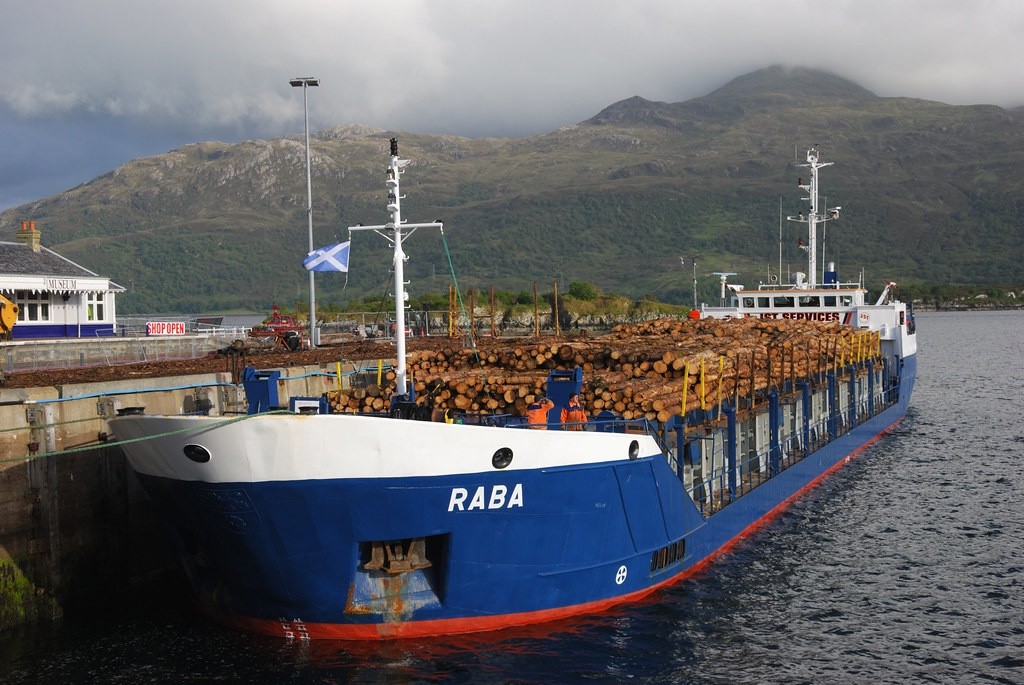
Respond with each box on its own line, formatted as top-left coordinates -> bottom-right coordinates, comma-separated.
302,241 -> 350,273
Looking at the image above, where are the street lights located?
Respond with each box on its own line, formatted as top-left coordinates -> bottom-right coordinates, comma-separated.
289,75 -> 323,348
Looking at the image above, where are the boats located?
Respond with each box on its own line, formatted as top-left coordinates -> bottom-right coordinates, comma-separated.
110,131 -> 919,646
